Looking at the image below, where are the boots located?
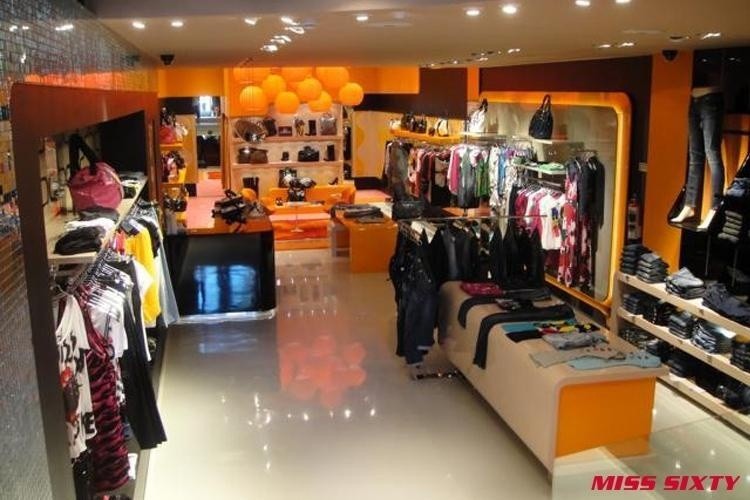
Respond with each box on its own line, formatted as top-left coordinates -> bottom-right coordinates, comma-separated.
305,120 -> 317,136
281,152 -> 289,161
324,145 -> 335,160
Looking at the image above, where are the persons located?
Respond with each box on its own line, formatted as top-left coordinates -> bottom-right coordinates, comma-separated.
668,87 -> 726,228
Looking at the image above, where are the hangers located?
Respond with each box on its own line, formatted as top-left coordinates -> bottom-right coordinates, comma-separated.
52,196 -> 166,321
383,137 -> 458,162
397,215 -> 548,249
452,141 -> 533,168
514,183 -> 569,202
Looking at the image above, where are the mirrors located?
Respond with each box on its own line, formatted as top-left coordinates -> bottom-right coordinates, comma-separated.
159,95 -> 226,197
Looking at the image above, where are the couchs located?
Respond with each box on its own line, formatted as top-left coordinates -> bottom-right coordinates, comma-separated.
436,279 -> 671,483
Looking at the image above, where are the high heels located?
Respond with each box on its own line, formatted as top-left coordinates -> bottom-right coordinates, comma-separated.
276,198 -> 279,205
328,178 -> 338,185
279,198 -> 283,206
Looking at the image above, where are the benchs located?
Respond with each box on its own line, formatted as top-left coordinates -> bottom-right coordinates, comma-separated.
329,202 -> 398,273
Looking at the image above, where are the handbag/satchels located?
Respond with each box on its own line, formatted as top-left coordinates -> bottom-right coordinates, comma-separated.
159,112 -> 177,144
528,95 -> 553,139
222,188 -> 243,205
262,117 -> 277,137
170,114 -> 183,143
433,116 -> 450,137
278,168 -> 298,188
415,112 -> 426,133
68,133 -> 124,211
468,98 -> 489,133
162,193 -> 171,209
318,112 -> 337,136
211,203 -> 246,217
286,180 -> 306,201
390,119 -> 401,130
401,112 -> 415,131
391,194 -> 425,221
250,149 -> 267,164
237,148 -> 257,164
243,176 -> 259,192
172,195 -> 186,212
298,146 -> 320,162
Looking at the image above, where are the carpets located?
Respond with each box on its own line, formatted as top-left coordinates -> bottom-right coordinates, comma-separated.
272,217 -> 333,251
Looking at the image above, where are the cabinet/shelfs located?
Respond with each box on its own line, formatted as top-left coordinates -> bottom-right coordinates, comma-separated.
11,82 -> 169,500
607,270 -> 750,437
226,99 -> 349,199
665,46 -> 750,302
